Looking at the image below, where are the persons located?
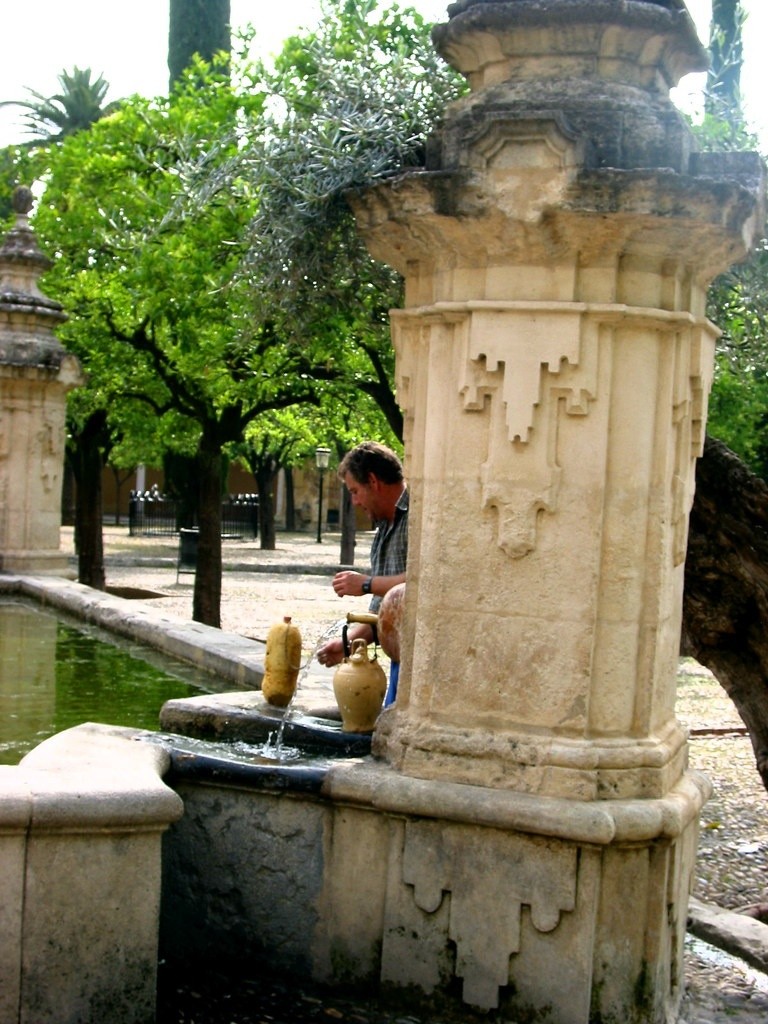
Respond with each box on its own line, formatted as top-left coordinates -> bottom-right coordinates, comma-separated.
317,441 -> 410,709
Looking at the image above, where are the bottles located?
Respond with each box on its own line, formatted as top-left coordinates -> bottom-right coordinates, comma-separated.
332,638 -> 388,733
261,615 -> 302,710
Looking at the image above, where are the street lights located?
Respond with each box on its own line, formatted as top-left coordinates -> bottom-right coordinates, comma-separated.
314,446 -> 332,543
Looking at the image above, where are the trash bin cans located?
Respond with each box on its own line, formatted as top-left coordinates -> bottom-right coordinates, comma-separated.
175,525 -> 201,566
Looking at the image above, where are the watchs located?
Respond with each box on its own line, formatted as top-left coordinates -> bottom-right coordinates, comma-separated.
362,576 -> 373,594
